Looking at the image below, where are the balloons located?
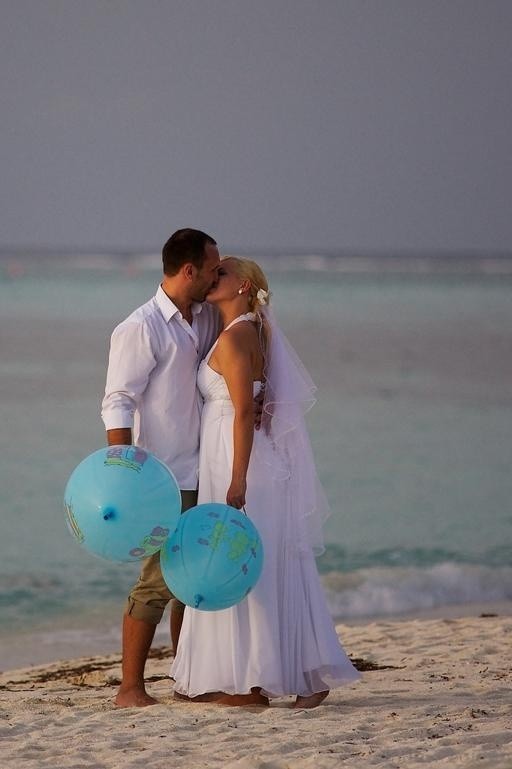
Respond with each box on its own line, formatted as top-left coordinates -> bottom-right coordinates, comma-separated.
160,503 -> 265,611
62,443 -> 184,563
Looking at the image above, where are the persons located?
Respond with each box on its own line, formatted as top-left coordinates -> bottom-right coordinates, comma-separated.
166,255 -> 364,711
100,226 -> 271,708
